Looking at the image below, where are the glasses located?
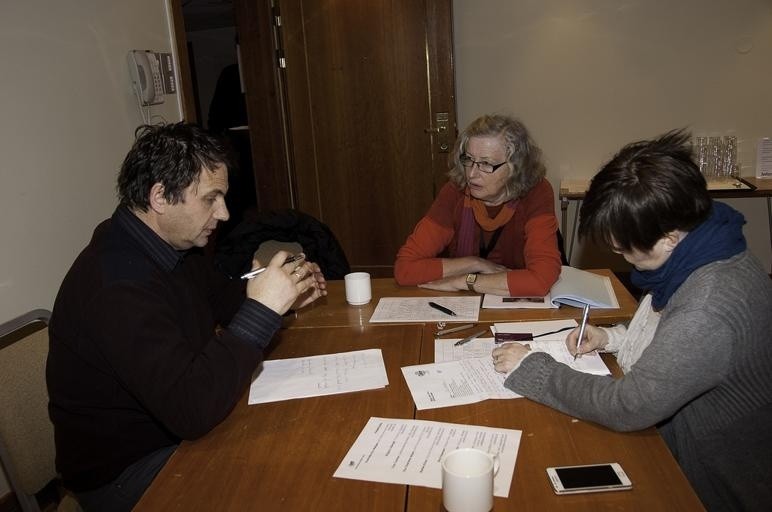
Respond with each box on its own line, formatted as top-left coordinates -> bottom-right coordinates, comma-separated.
460,152 -> 510,174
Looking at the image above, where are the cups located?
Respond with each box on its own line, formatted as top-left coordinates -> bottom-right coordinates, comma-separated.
347,304 -> 374,333
344,272 -> 372,306
695,136 -> 737,183
440,448 -> 495,512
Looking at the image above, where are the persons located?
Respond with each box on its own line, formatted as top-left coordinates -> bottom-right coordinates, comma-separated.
392,113 -> 566,298
491,129 -> 772,512
45,118 -> 329,512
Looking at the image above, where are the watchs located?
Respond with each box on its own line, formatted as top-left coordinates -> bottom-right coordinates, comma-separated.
466,271 -> 477,293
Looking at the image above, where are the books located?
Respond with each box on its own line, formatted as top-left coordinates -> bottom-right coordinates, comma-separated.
481,265 -> 620,310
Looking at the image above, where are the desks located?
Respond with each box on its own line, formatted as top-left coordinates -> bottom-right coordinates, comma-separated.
560,175 -> 772,265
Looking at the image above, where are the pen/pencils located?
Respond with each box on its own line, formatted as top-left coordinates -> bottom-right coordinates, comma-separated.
430,301 -> 456,316
571,304 -> 590,365
241,254 -> 303,280
454,329 -> 489,345
433,323 -> 478,336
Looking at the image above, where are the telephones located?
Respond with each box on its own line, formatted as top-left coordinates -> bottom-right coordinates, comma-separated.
127,49 -> 165,106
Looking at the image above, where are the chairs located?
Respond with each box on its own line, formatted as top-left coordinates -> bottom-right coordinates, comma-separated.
0,306 -> 85,511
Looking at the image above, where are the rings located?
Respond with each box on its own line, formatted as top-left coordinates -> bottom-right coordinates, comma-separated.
295,272 -> 305,280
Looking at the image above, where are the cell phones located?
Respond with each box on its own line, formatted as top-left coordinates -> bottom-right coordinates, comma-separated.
545,462 -> 633,495
